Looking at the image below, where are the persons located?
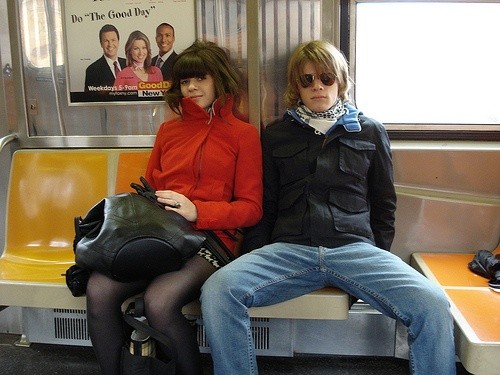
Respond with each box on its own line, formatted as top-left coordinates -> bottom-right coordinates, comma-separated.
84,23 -> 128,93
151,22 -> 179,82
110,31 -> 163,91
85,40 -> 263,375
200,36 -> 457,375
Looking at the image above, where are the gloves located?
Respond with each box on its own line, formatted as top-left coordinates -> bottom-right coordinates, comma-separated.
130,175 -> 165,210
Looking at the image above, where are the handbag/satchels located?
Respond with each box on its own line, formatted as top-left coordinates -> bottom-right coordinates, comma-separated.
75,193 -> 209,277
118,293 -> 179,375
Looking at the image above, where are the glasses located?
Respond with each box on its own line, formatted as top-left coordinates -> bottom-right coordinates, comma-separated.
296,71 -> 337,88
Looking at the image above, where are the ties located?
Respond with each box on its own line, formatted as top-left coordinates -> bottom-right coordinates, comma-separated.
113,61 -> 120,78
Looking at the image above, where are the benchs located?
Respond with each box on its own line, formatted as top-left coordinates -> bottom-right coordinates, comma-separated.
409,240 -> 500,375
0,147 -> 356,320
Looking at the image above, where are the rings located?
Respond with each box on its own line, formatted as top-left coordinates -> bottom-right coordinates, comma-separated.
174,200 -> 181,208
